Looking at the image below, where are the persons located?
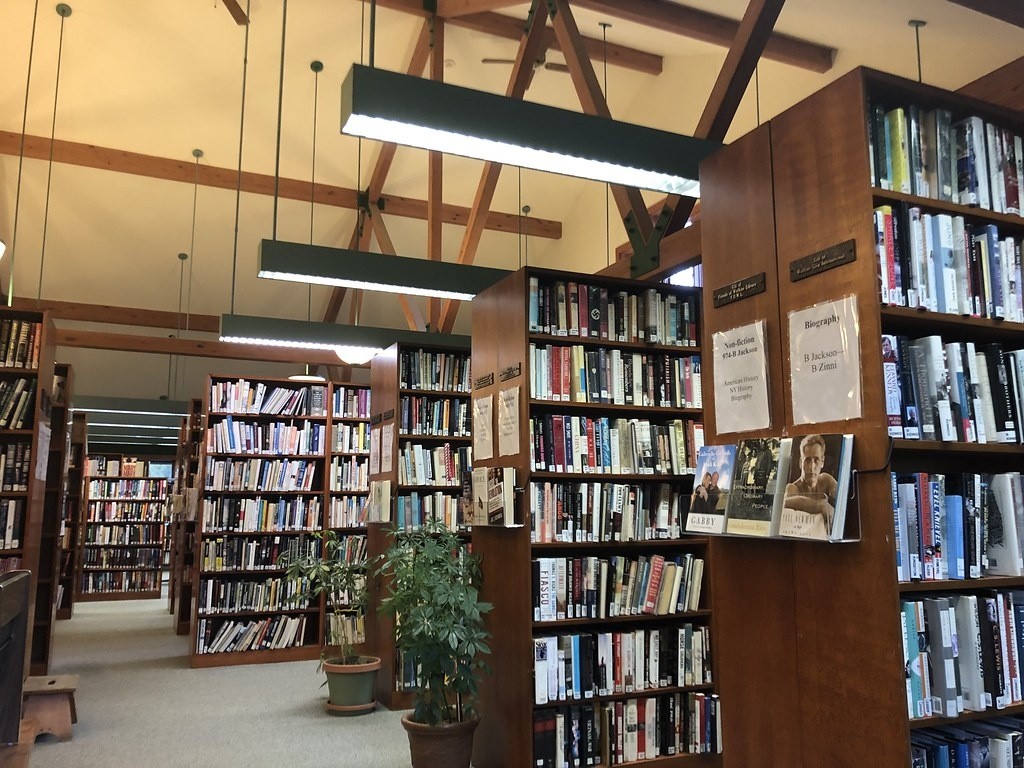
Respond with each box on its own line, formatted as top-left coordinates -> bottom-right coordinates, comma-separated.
882,337 -> 896,362
739,440 -> 774,506
783,435 -> 838,533
691,471 -> 722,514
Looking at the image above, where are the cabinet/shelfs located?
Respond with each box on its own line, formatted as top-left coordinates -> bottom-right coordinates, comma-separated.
187,372 -> 370,670
696,63 -> 1024,768
168,397 -> 202,637
370,341 -> 474,711
471,264 -> 723,768
0,303 -> 88,745
74,476 -> 169,603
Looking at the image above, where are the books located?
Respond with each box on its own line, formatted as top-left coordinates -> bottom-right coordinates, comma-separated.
0,318 -> 42,577
368,480 -> 391,522
198,574 -> 367,654
866,98 -> 1024,768
779,434 -> 854,540
395,348 -> 472,692
471,466 -> 516,525
211,379 -> 371,419
204,415 -> 370,492
528,278 -> 703,475
726,437 -> 794,537
685,445 -> 739,536
529,481 -> 723,768
54,445 -> 76,609
203,496 -> 369,572
76,480 -> 166,593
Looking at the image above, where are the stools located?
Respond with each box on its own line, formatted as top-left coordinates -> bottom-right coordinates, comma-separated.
18,675 -> 80,745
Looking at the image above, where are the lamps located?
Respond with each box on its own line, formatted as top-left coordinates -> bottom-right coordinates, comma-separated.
340,0 -> 728,200
256,0 -> 518,303
217,0 -> 472,358
288,61 -> 330,381
332,0 -> 380,365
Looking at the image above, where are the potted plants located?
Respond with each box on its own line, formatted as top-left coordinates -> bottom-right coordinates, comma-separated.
371,515 -> 483,768
276,530 -> 383,718
